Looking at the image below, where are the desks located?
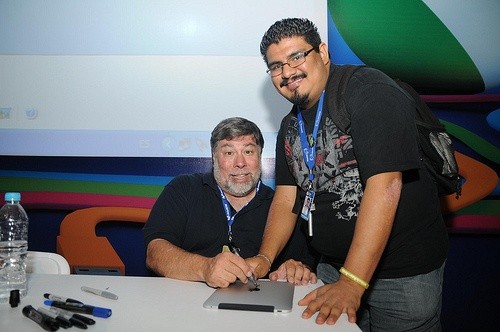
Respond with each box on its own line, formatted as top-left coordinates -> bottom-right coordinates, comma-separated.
0,274 -> 363,332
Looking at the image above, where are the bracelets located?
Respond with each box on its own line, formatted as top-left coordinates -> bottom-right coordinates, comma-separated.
339,266 -> 369,289
255,253 -> 272,268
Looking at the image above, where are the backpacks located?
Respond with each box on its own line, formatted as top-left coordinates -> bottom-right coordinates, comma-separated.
282,65 -> 466,214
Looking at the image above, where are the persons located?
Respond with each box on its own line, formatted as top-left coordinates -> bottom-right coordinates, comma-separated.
244,18 -> 449,332
145,117 -> 318,285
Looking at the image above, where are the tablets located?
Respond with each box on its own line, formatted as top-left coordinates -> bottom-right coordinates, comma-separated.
203,279 -> 296,312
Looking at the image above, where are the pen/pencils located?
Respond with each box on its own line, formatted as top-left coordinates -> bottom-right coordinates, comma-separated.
80,285 -> 118,300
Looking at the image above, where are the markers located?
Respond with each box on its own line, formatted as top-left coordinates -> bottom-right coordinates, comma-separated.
231,246 -> 258,287
22,293 -> 112,332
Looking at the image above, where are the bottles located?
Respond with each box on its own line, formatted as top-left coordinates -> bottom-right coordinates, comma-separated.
1,192 -> 29,299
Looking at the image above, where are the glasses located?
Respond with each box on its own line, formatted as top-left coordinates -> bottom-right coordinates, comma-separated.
266,47 -> 318,77
